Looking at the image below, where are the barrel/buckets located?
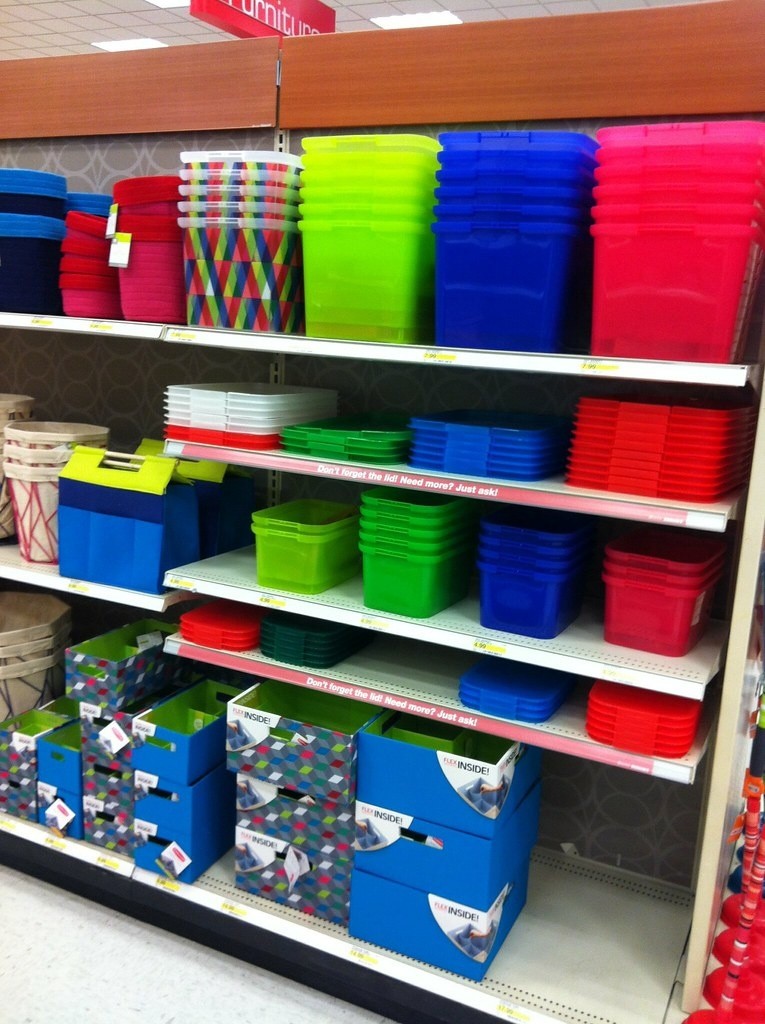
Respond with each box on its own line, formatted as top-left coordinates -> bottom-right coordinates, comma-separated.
1,390 -> 37,539
2,420 -> 110,567
0,590 -> 71,722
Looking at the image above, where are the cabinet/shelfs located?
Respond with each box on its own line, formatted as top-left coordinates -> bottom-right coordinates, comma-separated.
1,1 -> 765,1024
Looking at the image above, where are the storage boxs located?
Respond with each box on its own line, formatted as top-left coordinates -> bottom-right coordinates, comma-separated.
0,118 -> 765,983
